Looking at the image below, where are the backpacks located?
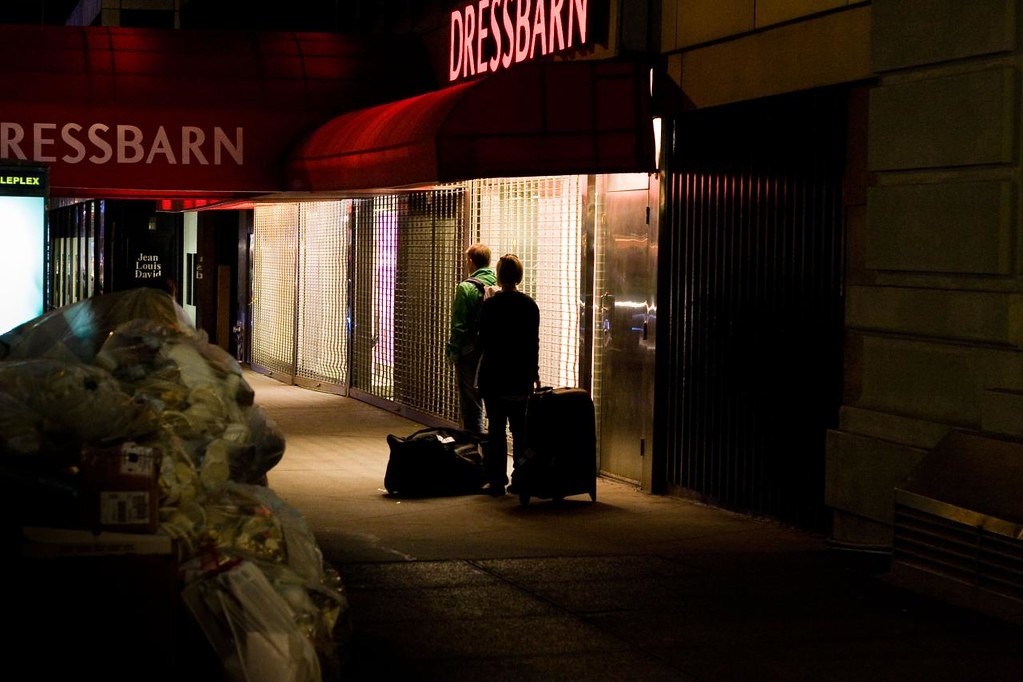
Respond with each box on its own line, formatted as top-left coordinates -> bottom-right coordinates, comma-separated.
464,276 -> 501,301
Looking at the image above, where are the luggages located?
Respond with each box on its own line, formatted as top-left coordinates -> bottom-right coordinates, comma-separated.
519,386 -> 597,506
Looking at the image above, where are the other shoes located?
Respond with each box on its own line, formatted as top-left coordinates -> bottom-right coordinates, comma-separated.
482,483 -> 505,497
507,478 -> 521,495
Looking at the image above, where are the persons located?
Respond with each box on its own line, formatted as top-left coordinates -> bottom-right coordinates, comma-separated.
446,243 -> 501,433
146,277 -> 180,302
474,254 -> 542,495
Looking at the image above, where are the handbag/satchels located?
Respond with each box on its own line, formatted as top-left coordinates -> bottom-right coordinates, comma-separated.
384,425 -> 491,498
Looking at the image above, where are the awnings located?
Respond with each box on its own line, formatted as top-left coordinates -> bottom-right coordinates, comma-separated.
0,24 -> 667,192
